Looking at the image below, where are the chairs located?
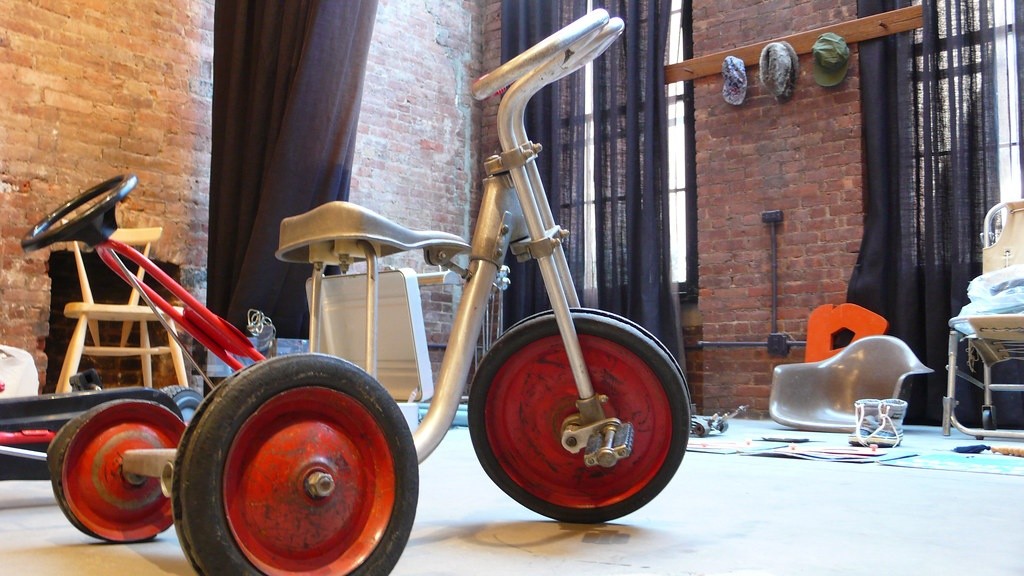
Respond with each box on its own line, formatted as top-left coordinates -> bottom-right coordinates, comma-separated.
942,198 -> 1024,438
768,335 -> 935,431
54,227 -> 189,395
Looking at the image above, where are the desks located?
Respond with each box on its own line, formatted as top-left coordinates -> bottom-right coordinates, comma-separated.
302,264 -> 512,404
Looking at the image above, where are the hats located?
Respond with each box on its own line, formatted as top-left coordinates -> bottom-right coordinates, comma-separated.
722,55 -> 747,105
811,33 -> 849,87
759,40 -> 800,102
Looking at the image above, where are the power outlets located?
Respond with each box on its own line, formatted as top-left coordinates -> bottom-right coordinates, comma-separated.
766,333 -> 789,357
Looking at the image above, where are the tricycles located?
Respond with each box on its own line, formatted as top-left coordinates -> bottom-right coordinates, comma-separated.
0,171 -> 270,483
45,9 -> 693,576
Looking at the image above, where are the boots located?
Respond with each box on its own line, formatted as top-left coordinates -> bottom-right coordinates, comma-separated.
867,399 -> 908,448
848,398 -> 879,446
